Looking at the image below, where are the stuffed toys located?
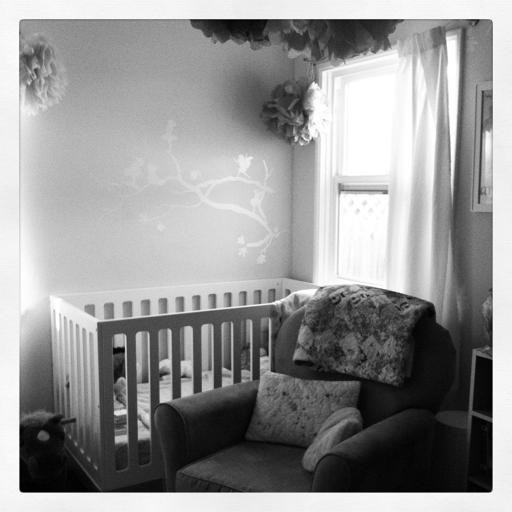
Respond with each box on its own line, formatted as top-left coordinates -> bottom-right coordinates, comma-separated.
19,410 -> 66,493
158,359 -> 193,379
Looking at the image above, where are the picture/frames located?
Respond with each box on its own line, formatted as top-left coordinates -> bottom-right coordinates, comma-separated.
470,80 -> 492,214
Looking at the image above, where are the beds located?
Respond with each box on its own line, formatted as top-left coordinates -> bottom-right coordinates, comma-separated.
50,278 -> 362,492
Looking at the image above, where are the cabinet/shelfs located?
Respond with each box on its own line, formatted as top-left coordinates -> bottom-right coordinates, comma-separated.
467,348 -> 493,492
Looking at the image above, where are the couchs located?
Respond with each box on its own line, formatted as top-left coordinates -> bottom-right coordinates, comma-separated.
154,285 -> 455,491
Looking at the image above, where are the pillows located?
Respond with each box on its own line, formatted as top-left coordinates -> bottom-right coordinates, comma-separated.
244,370 -> 362,444
